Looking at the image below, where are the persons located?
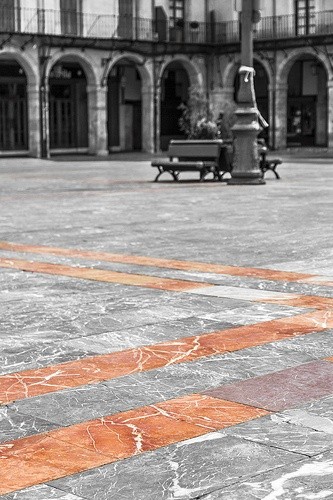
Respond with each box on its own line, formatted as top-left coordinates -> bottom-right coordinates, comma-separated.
215,112 -> 225,139
195,110 -> 221,183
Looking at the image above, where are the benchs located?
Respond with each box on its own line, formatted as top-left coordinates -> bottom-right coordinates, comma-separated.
257,148 -> 282,180
151,140 -> 225,181
